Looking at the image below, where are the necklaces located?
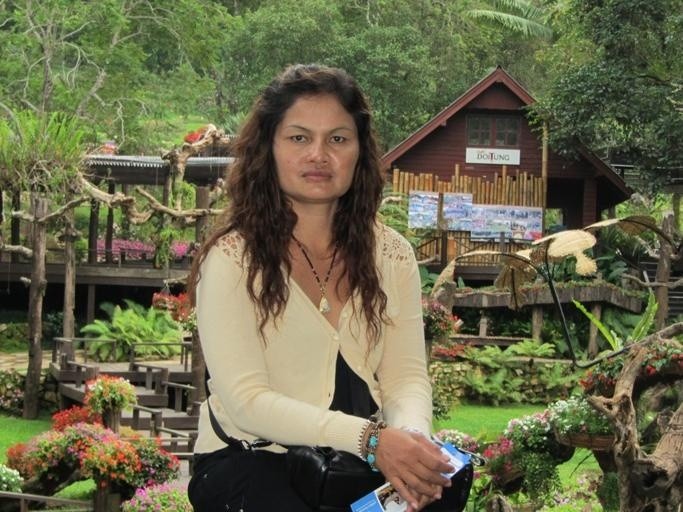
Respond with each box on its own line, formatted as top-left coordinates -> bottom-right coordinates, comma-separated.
290,232 -> 341,314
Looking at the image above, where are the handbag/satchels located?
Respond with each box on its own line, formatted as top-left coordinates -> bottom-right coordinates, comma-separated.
187,443 -> 373,512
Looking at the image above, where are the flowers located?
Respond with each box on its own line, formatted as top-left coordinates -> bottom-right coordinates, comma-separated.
0,374 -> 194,511
436,395 -> 614,509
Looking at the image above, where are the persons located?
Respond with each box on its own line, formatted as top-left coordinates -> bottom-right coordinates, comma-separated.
59,227 -> 81,242
188,64 -> 473,512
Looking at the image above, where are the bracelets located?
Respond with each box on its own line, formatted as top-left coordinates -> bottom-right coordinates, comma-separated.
365,421 -> 387,472
